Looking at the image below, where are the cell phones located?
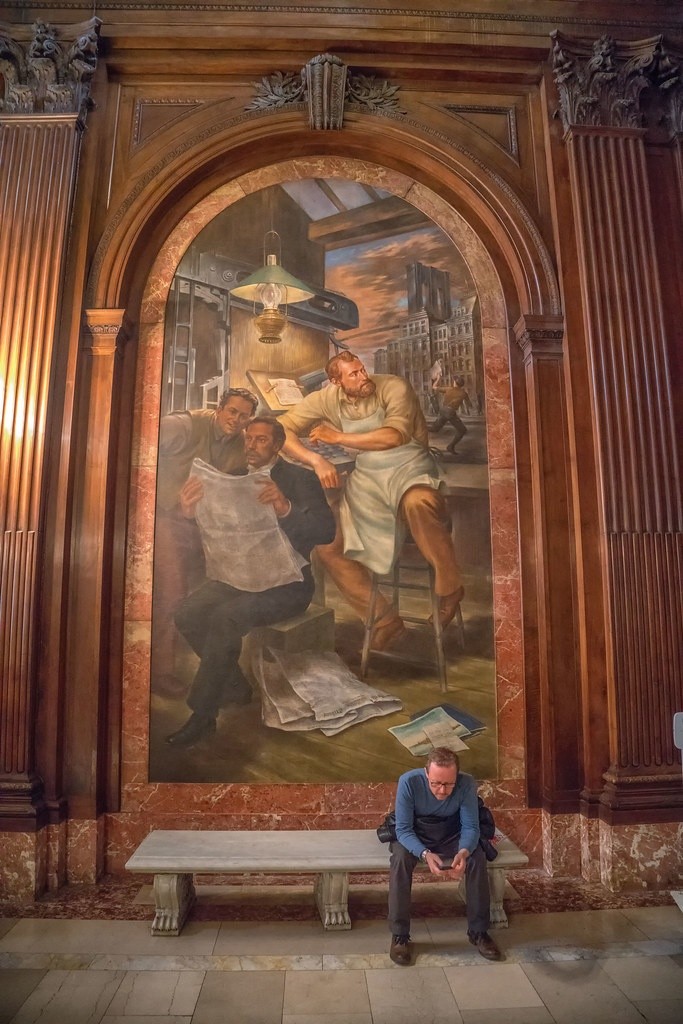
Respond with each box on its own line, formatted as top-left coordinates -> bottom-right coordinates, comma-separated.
440,866 -> 453,870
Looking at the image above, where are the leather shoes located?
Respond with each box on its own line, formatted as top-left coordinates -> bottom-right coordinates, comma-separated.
469,931 -> 502,959
390,933 -> 412,965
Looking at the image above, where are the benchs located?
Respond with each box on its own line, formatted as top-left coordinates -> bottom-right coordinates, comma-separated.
126,829 -> 530,937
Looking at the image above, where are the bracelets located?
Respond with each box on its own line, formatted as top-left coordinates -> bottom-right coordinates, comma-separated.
422,849 -> 431,864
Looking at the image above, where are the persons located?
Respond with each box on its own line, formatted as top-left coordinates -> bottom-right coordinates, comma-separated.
388,747 -> 503,965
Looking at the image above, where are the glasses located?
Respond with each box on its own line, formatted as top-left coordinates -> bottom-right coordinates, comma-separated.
425,766 -> 458,788
